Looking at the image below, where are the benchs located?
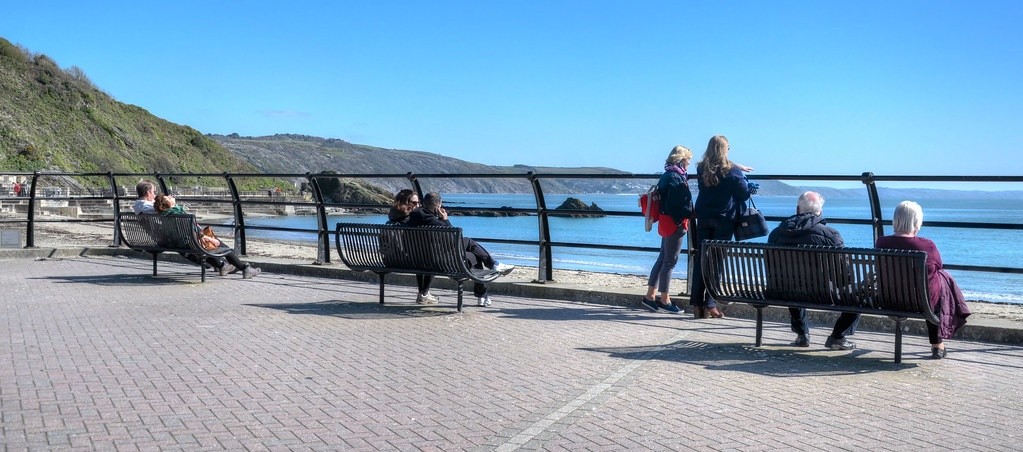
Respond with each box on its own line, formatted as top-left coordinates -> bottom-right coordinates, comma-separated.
336,222 -> 499,312
700,239 -> 939,365
117,212 -> 234,282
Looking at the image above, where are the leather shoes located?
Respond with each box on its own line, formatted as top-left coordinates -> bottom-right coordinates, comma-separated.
795,334 -> 810,346
830,336 -> 856,349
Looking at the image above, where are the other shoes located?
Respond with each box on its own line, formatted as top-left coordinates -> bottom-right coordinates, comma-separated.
214,267 -> 220,271
932,346 -> 946,359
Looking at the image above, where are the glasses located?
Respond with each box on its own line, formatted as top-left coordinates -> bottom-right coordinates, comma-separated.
412,201 -> 419,205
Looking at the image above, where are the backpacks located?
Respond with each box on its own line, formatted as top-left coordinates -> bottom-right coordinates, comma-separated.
639,184 -> 661,231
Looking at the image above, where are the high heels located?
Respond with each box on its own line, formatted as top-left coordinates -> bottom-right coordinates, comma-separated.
694,305 -> 705,318
704,305 -> 723,318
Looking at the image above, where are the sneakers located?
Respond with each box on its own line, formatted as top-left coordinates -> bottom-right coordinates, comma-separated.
219,261 -> 236,275
642,297 -> 660,311
491,262 -> 515,275
417,291 -> 439,304
478,296 -> 491,306
243,263 -> 261,278
658,302 -> 684,314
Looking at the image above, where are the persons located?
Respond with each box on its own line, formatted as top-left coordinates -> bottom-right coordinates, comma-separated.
133,181 -> 262,280
14,181 -> 28,197
690,135 -> 754,319
381,188 -> 515,305
268,188 -> 280,198
640,145 -> 695,315
767,191 -> 863,350
875,200 -> 973,359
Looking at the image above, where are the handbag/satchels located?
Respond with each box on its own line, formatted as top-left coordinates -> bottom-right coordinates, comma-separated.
197,227 -> 217,250
733,197 -> 769,240
